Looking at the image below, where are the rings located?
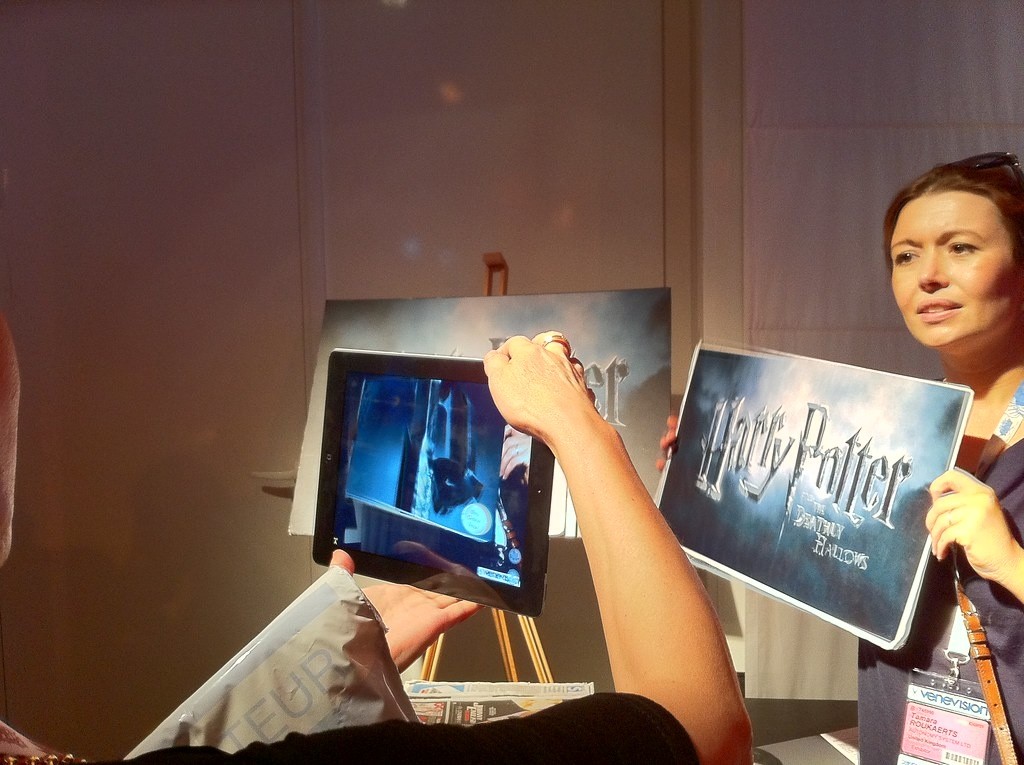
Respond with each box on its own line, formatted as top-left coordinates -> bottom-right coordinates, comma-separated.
515,443 -> 519,455
947,509 -> 955,528
541,335 -> 572,356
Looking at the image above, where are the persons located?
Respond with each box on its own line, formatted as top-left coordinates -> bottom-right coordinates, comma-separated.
1,312 -> 755,765
498,422 -> 532,545
653,151 -> 1024,765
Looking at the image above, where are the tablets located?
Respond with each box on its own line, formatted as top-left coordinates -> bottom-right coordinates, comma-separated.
312,347 -> 556,618
